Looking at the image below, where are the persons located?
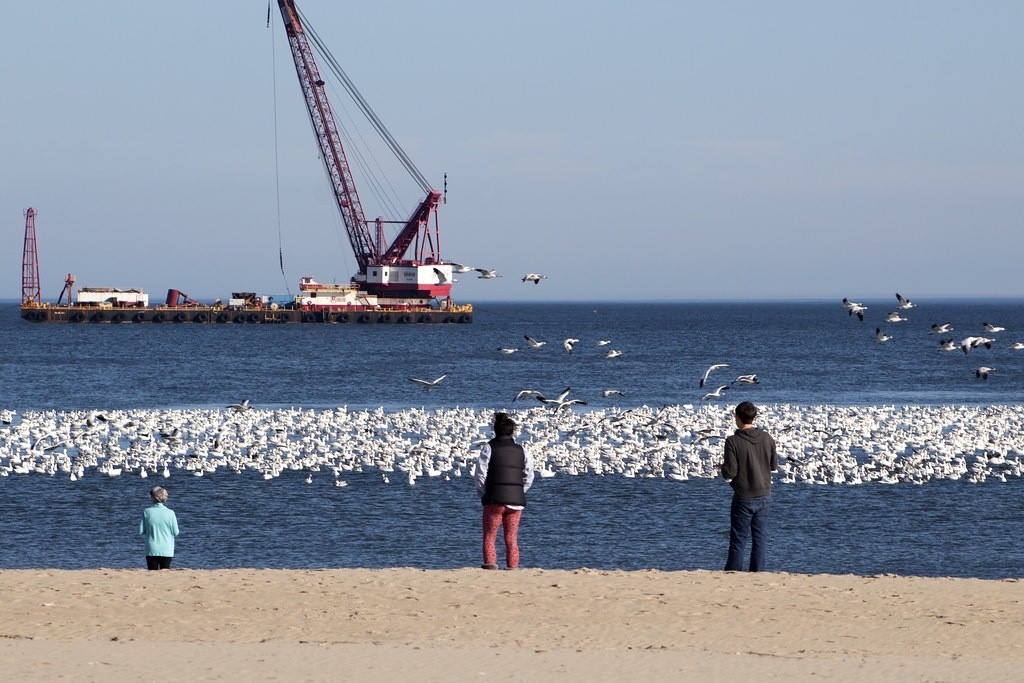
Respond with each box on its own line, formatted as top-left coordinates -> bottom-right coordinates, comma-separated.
474,418 -> 535,570
720,400 -> 779,572
139,486 -> 179,570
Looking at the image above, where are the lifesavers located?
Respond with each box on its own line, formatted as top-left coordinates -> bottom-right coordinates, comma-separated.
283,313 -> 291,322
116,313 -> 126,323
402,315 -> 411,323
422,314 -> 472,323
75,312 -> 84,322
237,314 -> 245,323
256,300 -> 263,308
95,312 -> 105,321
157,313 -> 166,322
38,312 -> 47,321
251,313 -> 260,322
197,313 -> 207,321
383,315 -> 391,323
361,314 -> 370,323
29,311 -> 37,319
220,313 -> 229,322
306,314 -> 316,322
340,314 -> 349,322
137,313 -> 146,321
177,313 -> 187,322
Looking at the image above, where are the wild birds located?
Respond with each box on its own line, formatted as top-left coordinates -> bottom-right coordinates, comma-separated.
564,339 -> 579,354
408,371 -> 449,386
884,312 -> 908,322
982,322 -> 1005,333
895,293 -> 918,309
700,363 -> 730,387
596,340 -> 610,346
0,366 -> 1024,497
605,350 -> 623,359
937,336 -> 996,354
433,268 -> 459,287
840,298 -> 868,321
522,274 -> 550,285
873,328 -> 893,342
524,335 -> 546,349
927,322 -> 954,335
1008,343 -> 1024,350
497,348 -> 521,356
476,269 -> 503,279
443,262 -> 476,273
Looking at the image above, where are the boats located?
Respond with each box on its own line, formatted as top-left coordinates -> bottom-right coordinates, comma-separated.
18,206 -> 473,324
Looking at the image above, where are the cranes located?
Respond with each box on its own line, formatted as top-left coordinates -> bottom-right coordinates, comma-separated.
262,0 -> 459,310
